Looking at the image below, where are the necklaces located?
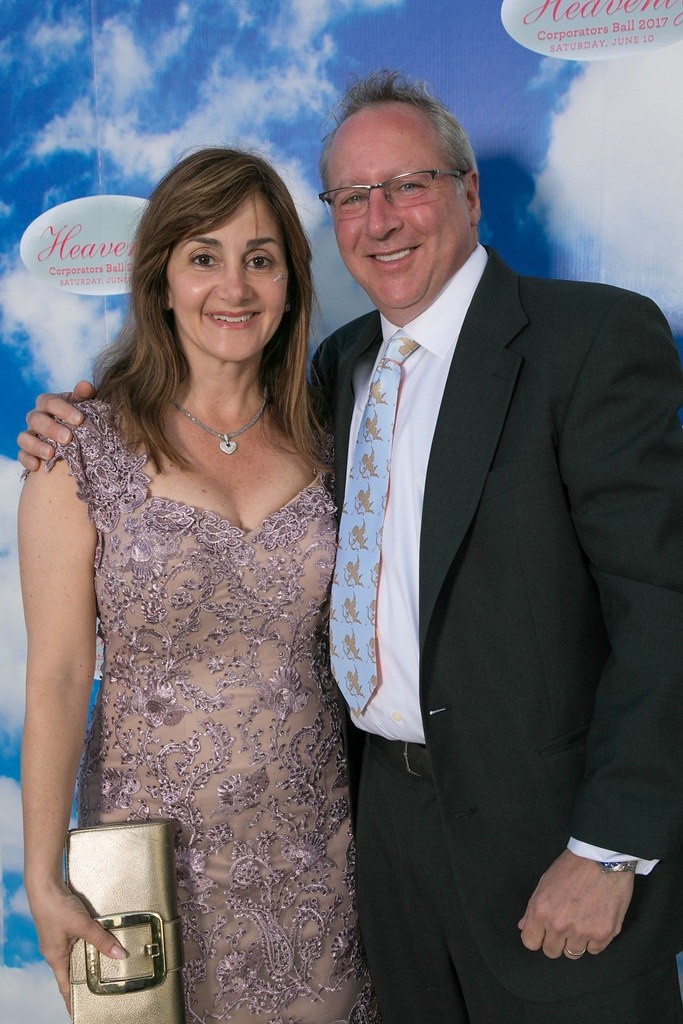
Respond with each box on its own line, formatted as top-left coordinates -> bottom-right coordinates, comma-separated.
169,383 -> 268,455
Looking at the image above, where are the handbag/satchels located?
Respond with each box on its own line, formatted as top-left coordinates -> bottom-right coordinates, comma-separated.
67,818 -> 184,1024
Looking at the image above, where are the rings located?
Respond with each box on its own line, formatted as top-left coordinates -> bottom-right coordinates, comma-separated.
564,946 -> 587,957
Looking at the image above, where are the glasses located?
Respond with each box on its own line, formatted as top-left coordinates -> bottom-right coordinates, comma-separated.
318,167 -> 463,220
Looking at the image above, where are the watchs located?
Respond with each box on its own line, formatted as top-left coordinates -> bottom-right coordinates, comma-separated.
596,862 -> 637,873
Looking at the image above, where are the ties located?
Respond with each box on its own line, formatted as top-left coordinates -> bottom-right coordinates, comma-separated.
329,330 -> 420,718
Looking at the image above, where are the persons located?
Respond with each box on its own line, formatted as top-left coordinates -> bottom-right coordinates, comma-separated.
17,149 -> 381,1024
16,69 -> 683,1024
